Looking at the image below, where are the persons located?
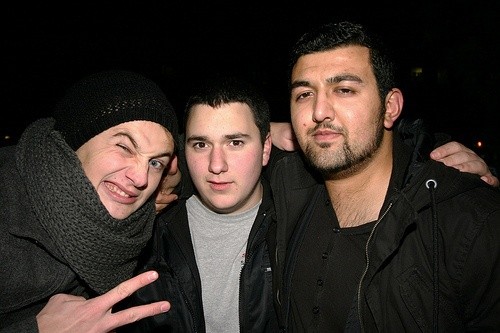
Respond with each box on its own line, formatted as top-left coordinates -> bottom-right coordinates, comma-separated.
0,64 -> 296,333
127,75 -> 500,332
156,19 -> 500,333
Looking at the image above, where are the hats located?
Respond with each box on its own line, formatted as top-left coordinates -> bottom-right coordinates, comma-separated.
54,71 -> 178,152
